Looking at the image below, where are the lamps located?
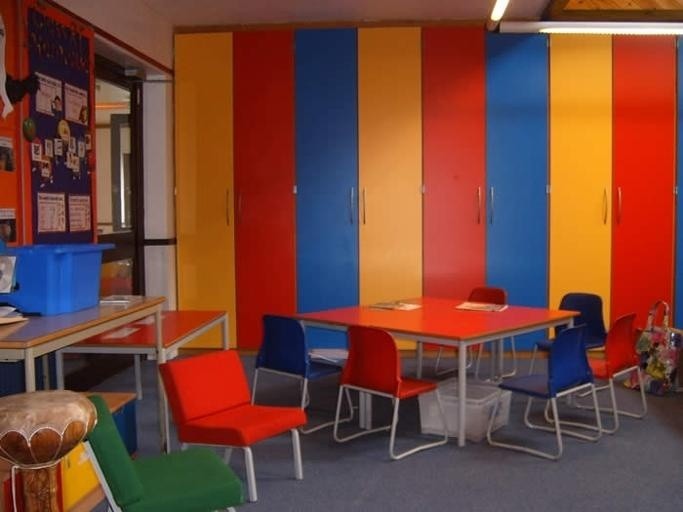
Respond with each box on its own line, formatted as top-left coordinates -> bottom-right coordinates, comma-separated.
485,0 -> 683,36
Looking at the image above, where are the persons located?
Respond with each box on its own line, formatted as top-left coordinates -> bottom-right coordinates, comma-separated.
51,96 -> 63,119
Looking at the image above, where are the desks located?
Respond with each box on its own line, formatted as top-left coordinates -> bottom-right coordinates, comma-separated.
297,297 -> 580,449
54,309 -> 230,400
0,295 -> 169,454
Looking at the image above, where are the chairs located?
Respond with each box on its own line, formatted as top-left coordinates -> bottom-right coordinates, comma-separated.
333,323 -> 449,460
85,394 -> 245,512
528,291 -> 605,375
486,322 -> 603,461
250,313 -> 354,434
434,286 -> 518,380
158,350 -> 309,503
545,313 -> 648,435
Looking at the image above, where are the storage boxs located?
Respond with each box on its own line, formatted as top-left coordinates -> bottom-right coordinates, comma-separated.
0,243 -> 116,316
418,384 -> 513,444
76,392 -> 139,456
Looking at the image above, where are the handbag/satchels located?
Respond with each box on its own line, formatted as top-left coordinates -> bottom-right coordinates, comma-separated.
624,301 -> 683,397
